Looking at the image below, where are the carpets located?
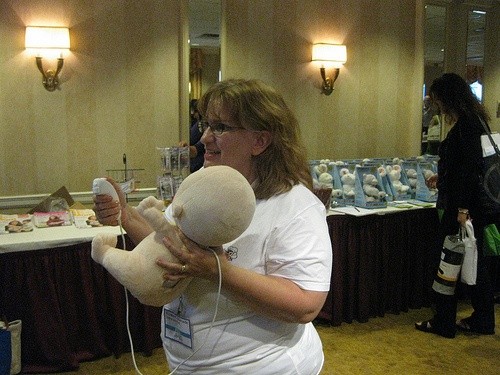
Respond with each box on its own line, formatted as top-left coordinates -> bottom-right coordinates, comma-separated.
61,297 -> 500,375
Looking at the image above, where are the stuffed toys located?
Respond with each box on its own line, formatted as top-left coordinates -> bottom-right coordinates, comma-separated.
424,168 -> 438,195
314,164 -> 342,197
91,166 -> 256,307
320,156 -> 437,177
363,173 -> 386,202
407,168 -> 418,191
386,165 -> 409,191
340,168 -> 356,195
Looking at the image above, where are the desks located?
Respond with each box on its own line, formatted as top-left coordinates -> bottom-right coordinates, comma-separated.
326,199 -> 438,326
0,208 -> 127,359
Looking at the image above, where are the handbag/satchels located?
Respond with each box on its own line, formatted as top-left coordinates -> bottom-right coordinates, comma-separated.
0,310 -> 22,375
432,219 -> 477,296
483,223 -> 500,257
458,109 -> 500,215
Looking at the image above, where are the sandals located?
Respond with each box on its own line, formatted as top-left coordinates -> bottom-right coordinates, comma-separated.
415,319 -> 455,339
456,319 -> 494,335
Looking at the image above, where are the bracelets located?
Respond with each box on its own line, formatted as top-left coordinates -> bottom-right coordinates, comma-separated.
458,209 -> 469,214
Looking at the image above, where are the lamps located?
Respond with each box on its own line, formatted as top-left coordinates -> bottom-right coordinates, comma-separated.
25,26 -> 71,92
312,43 -> 347,96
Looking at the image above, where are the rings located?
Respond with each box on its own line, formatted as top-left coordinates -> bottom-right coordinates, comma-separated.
181,266 -> 185,273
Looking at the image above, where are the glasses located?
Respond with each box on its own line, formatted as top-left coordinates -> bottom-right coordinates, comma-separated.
198,119 -> 263,136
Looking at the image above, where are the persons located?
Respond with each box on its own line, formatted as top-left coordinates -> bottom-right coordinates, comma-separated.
422,94 -> 436,135
415,72 -> 500,339
190,98 -> 206,173
90,79 -> 332,375
